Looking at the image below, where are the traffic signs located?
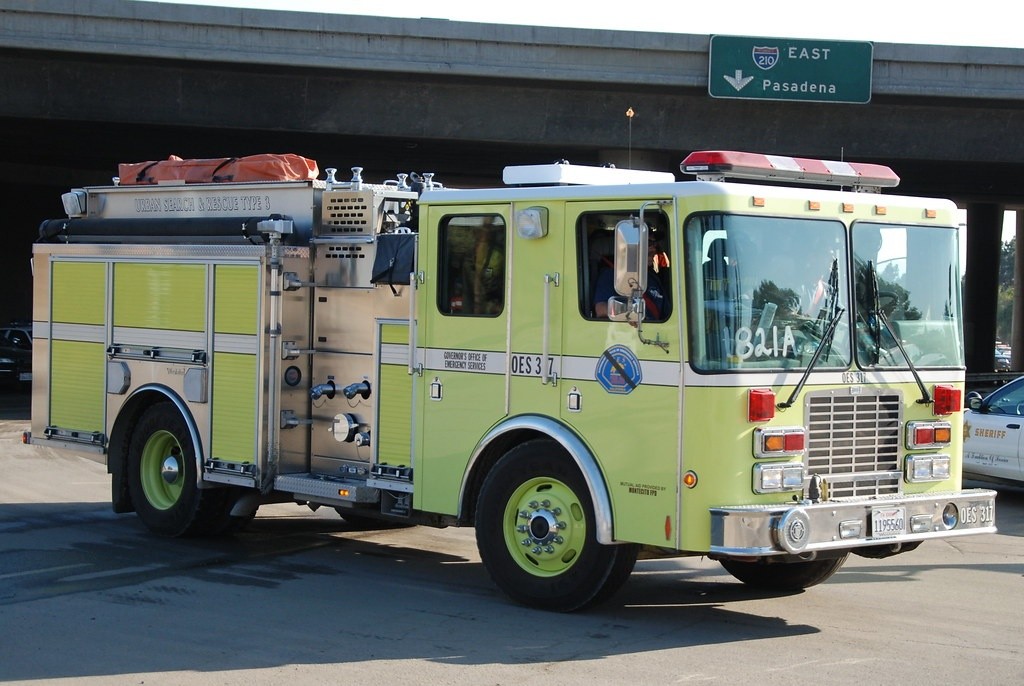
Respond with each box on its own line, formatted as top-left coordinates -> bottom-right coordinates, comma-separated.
706,34 -> 875,106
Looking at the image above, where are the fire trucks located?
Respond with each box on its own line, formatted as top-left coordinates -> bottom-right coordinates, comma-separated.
22,149 -> 1001,607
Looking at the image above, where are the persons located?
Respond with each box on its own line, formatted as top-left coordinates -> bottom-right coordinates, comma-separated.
595,227 -> 671,322
824,224 -> 922,337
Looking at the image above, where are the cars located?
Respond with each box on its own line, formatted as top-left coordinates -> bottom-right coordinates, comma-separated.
995,337 -> 1012,373
963,377 -> 1024,488
0,327 -> 33,382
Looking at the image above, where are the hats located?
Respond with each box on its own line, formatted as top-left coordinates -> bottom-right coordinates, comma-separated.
647,224 -> 665,241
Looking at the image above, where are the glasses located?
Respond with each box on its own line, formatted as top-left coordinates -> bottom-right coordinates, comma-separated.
649,239 -> 659,248
